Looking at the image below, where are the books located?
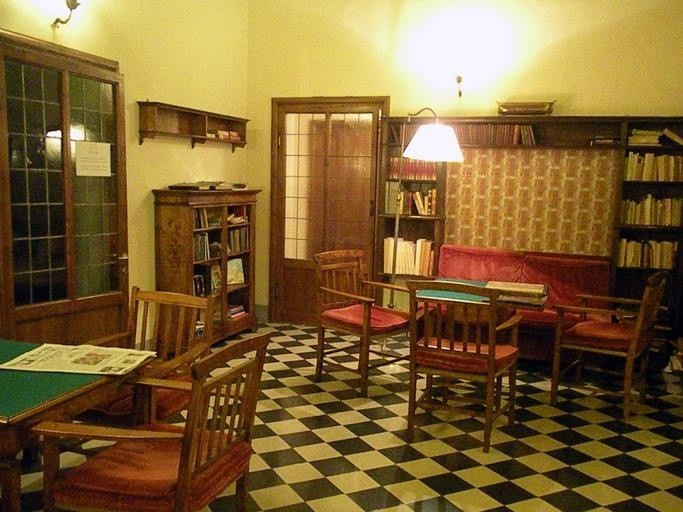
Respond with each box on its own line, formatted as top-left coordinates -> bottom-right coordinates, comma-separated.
384,158 -> 436,217
167,180 -> 249,191
205,129 -> 241,142
586,132 -> 619,146
388,123 -> 535,146
192,205 -> 250,336
381,236 -> 434,276
616,126 -> 683,271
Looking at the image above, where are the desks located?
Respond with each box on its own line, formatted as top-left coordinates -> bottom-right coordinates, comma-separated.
0,338 -> 150,512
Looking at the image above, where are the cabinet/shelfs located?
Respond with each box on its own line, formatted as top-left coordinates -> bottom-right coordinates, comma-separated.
151,188 -> 263,354
137,99 -> 250,154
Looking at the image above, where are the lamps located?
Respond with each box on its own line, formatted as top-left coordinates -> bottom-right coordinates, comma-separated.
386,109 -> 466,307
